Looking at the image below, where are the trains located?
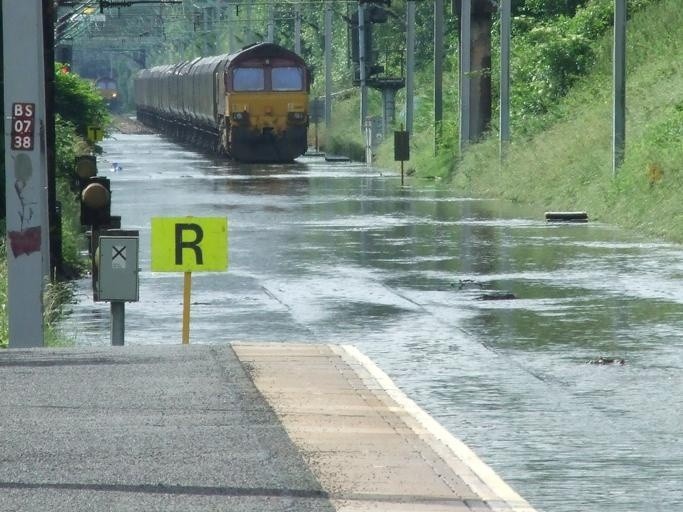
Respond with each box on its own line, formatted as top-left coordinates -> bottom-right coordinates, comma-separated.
132,40 -> 308,165
92,76 -> 121,109
